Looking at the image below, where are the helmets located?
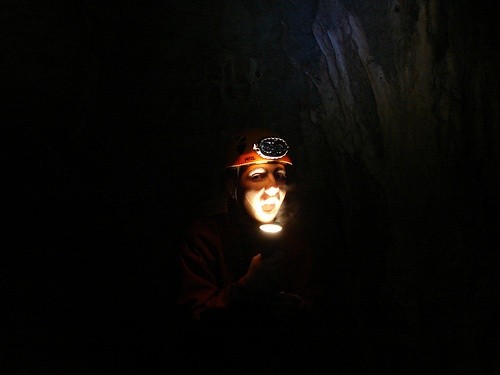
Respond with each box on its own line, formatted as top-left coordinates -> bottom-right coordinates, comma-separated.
226,127 -> 293,167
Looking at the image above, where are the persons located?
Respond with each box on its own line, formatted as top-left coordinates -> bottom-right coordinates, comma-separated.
174,128 -> 321,375
